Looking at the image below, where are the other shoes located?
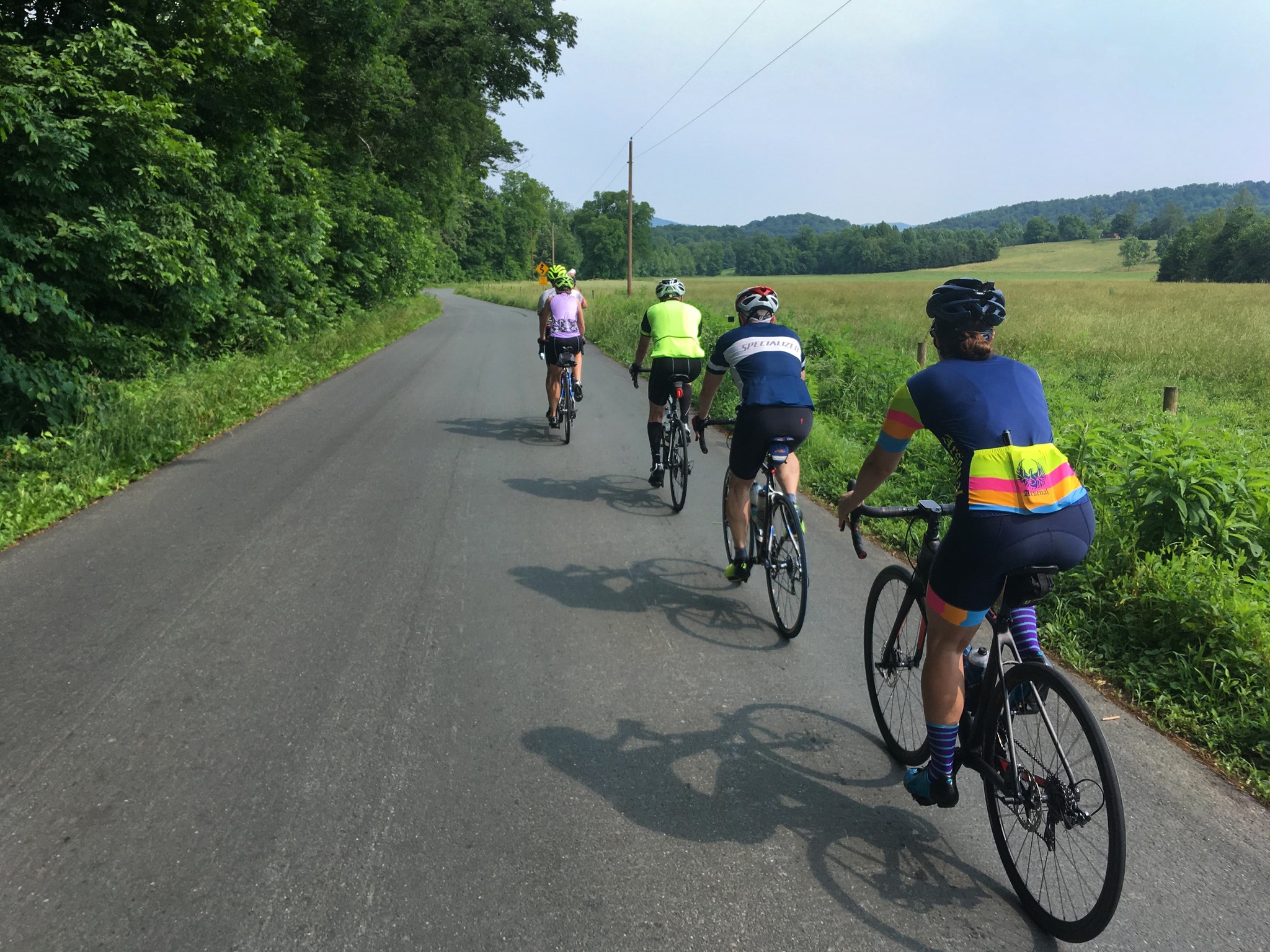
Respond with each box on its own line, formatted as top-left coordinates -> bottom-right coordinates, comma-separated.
550,416 -> 557,427
545,407 -> 551,418
571,371 -> 583,392
678,422 -> 691,447
648,461 -> 664,484
574,380 -> 583,402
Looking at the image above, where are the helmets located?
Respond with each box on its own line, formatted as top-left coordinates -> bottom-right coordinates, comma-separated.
735,285 -> 780,314
553,275 -> 574,288
926,277 -> 1007,327
546,264 -> 567,280
656,278 -> 685,298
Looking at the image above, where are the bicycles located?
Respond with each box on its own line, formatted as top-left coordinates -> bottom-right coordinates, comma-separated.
694,420 -> 810,639
538,339 -> 585,445
632,368 -> 695,511
839,500 -> 1128,945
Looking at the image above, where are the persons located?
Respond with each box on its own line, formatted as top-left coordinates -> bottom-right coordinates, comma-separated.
538,265 -> 588,427
629,279 -> 705,482
838,280 -> 1096,809
692,288 -> 815,581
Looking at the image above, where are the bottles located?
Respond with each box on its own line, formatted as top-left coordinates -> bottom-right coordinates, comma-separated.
662,405 -> 670,439
756,485 -> 767,530
749,482 -> 761,523
664,413 -> 671,446
964,646 -> 990,712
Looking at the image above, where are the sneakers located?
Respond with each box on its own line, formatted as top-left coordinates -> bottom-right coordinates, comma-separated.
723,556 -> 751,580
1013,650 -> 1053,702
784,502 -> 805,536
903,760 -> 961,809
